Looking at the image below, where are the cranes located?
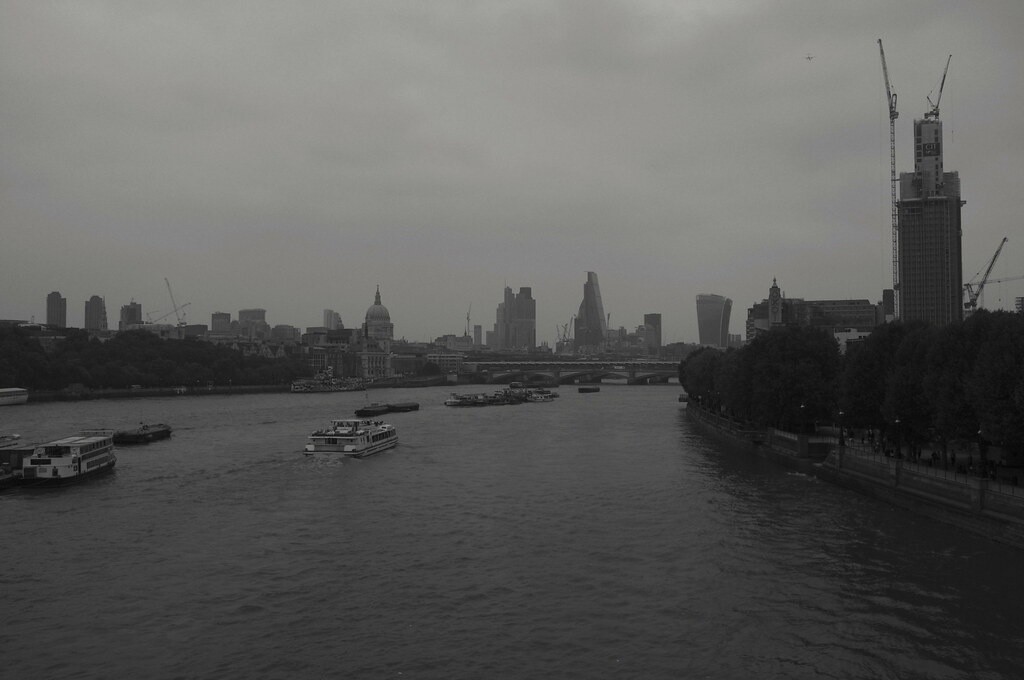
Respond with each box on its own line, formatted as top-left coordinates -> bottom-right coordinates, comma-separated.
962,236 -> 1007,312
874,37 -> 901,321
555,316 -> 575,354
923,53 -> 952,121
147,277 -> 192,328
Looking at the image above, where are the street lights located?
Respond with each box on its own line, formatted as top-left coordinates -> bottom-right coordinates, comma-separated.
895,420 -> 902,459
838,412 -> 846,446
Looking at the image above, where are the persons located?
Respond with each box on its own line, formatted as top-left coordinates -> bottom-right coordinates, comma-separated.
311,417 -> 383,435
840,426 -> 1024,489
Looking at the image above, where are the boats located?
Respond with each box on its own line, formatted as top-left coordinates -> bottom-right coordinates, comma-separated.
113,422 -> 172,446
0,387 -> 29,406
20,430 -> 117,490
354,401 -> 420,419
444,381 -> 601,407
290,365 -> 364,394
0,433 -> 41,494
301,419 -> 400,460
